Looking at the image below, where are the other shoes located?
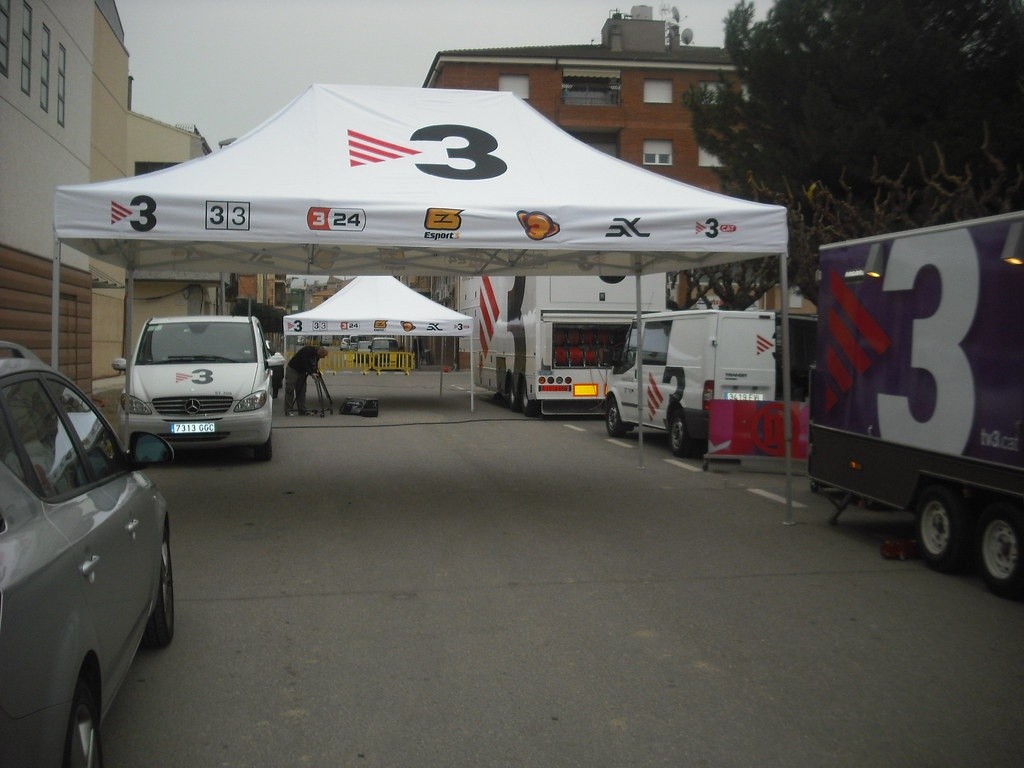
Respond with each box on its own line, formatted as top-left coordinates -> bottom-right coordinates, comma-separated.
287,413 -> 295,417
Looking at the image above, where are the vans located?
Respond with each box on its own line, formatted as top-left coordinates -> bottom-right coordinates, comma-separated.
604,309 -> 784,458
296,335 -> 305,345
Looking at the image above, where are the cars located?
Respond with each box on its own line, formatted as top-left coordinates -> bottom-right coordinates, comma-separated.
111,314 -> 288,463
341,333 -> 404,363
0,339 -> 179,768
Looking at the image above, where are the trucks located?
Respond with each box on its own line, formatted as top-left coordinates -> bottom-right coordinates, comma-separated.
804,209 -> 1024,604
318,333 -> 333,346
471,264 -> 668,418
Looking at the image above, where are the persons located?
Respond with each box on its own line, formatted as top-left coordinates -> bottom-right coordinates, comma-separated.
284,346 -> 329,416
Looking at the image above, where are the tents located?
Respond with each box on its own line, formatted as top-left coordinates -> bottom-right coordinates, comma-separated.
283,275 -> 473,413
51,81 -> 797,526
